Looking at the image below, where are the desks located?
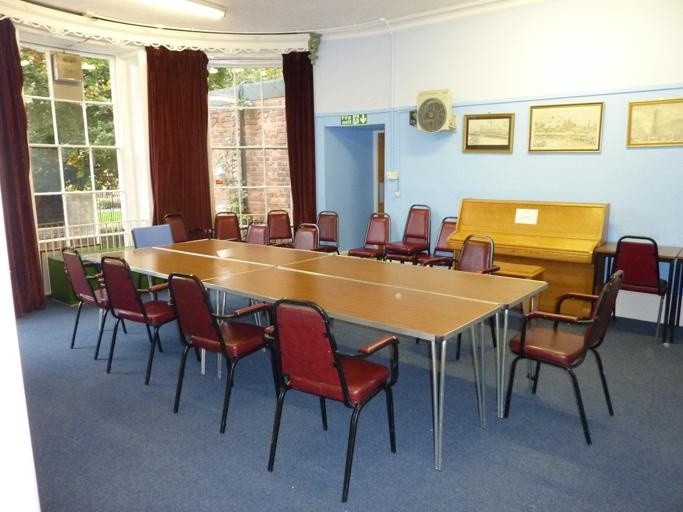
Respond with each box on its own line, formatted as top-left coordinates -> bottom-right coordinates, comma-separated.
83,234 -> 547,470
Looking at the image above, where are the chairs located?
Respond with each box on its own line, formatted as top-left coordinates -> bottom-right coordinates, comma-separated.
61,246 -> 153,361
100,256 -> 202,389
166,272 -> 276,434
132,225 -> 180,295
593,232 -> 681,346
416,235 -> 495,359
160,205 -> 461,300
266,297 -> 400,503
501,269 -> 625,447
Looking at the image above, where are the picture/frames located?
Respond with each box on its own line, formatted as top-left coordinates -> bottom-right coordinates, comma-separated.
525,100 -> 602,154
625,96 -> 682,149
461,113 -> 514,155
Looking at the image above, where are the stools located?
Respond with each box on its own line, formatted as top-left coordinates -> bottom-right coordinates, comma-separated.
493,257 -> 545,319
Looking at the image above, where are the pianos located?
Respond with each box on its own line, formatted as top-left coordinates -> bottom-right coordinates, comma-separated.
445,198 -> 610,320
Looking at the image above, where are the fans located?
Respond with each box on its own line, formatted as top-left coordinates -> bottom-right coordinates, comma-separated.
416,88 -> 455,135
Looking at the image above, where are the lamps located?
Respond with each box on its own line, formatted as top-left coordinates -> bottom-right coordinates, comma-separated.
152,1 -> 230,24
